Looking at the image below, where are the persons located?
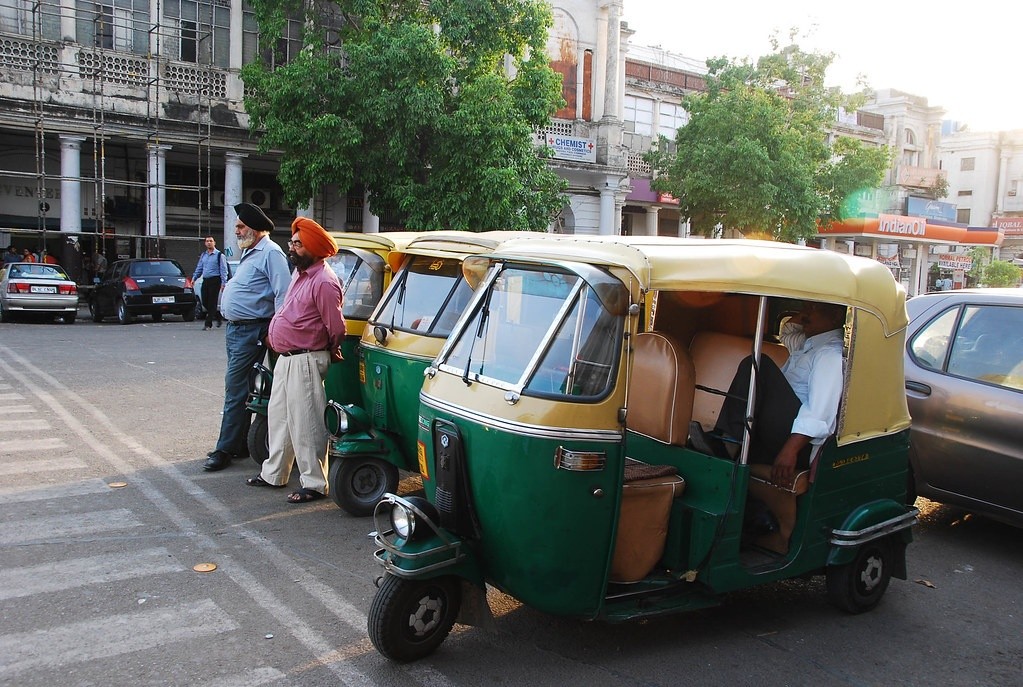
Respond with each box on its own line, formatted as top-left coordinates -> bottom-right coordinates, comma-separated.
190,235 -> 228,330
4,245 -> 60,265
941,275 -> 952,291
81,252 -> 107,281
245,215 -> 348,503
687,303 -> 847,538
330,254 -> 345,280
936,276 -> 942,291
203,203 -> 292,472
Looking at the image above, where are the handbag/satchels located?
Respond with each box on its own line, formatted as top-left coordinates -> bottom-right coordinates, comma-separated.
217,251 -> 232,281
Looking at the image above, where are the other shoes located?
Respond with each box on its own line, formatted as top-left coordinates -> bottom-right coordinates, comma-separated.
741,505 -> 778,535
216,314 -> 221,328
687,420 -> 730,460
202,325 -> 210,331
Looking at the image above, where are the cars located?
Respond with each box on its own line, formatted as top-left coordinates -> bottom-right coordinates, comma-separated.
88,258 -> 196,324
193,260 -> 241,319
0,263 -> 78,324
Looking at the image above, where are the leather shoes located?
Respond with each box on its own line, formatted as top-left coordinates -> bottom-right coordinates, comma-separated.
208,447 -> 250,458
203,449 -> 232,471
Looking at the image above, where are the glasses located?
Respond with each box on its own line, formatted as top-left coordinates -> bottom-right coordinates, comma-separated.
288,241 -> 302,249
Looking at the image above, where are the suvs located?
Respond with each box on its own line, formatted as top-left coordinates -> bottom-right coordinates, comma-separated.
905,288 -> 1023,528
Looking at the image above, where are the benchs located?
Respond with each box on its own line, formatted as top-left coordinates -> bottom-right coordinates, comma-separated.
670,331 -> 845,556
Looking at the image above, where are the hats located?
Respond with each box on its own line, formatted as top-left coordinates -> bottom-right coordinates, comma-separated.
291,216 -> 338,256
234,203 -> 275,232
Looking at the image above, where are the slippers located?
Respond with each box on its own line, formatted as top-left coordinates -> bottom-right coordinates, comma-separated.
286,488 -> 323,503
246,474 -> 279,486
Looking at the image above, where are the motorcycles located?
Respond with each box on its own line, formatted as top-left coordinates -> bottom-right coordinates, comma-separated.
244,231 -> 470,467
324,231 -> 540,518
365,233 -> 920,662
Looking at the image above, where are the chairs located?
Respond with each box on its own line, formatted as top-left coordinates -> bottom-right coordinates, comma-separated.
598,329 -> 694,580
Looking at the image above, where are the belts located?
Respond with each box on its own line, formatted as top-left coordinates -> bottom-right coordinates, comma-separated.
281,346 -> 328,358
228,317 -> 271,326
